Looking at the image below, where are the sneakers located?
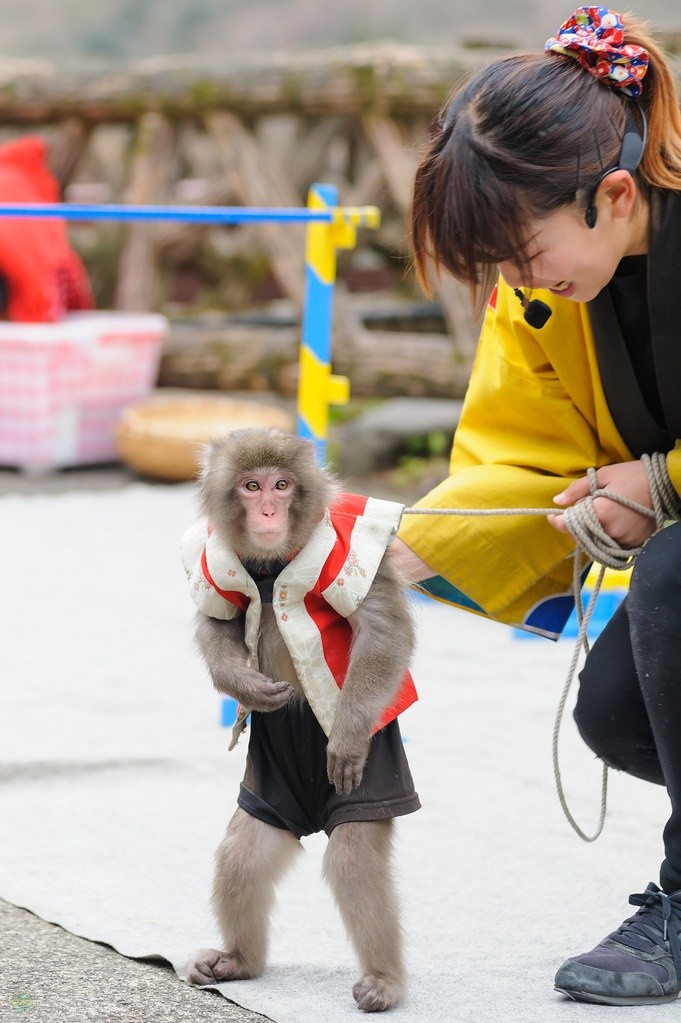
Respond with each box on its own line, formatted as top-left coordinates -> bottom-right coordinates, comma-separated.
555,884 -> 680,1007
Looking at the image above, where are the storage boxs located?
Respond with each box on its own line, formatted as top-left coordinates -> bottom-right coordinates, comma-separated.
0,309 -> 168,478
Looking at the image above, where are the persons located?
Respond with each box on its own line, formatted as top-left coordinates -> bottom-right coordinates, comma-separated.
383,3 -> 681,1020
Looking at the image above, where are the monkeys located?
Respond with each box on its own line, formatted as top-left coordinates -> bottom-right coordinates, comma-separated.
180,426 -> 432,1018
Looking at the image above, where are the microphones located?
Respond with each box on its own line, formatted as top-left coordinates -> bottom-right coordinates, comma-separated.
514,289 -> 551,329
